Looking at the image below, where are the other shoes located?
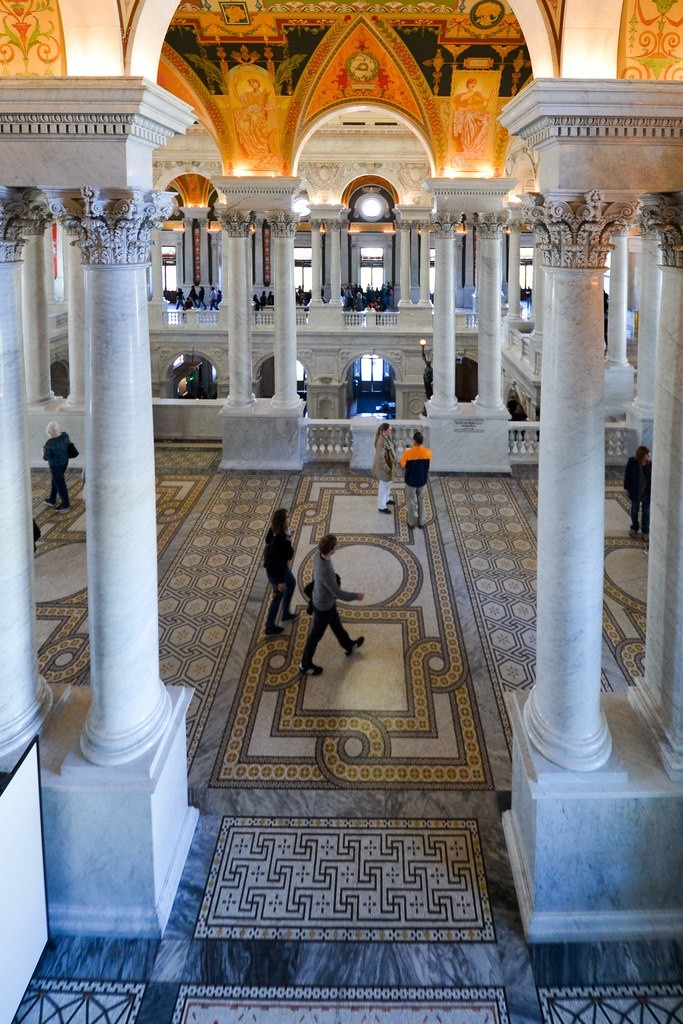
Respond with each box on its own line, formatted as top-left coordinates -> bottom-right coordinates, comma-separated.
387,500 -> 396,505
54,503 -> 71,512
641,532 -> 649,541
419,523 -> 423,529
264,624 -> 286,637
379,507 -> 391,514
631,530 -> 639,537
407,522 -> 414,529
281,611 -> 299,625
43,498 -> 55,507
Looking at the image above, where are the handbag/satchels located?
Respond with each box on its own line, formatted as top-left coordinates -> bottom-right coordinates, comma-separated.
66,442 -> 79,459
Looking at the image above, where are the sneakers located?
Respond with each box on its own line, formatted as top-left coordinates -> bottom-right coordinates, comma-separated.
345,636 -> 364,657
299,660 -> 323,675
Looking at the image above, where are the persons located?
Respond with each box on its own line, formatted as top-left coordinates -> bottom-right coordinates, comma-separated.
191,382 -> 208,400
604,290 -> 609,351
299,534 -> 365,676
526,286 -> 532,308
401,432 -> 432,529
372,423 -> 396,514
264,508 -> 299,635
176,281 -> 395,316
41,421 -> 71,513
624,445 -> 652,541
507,394 -> 528,442
